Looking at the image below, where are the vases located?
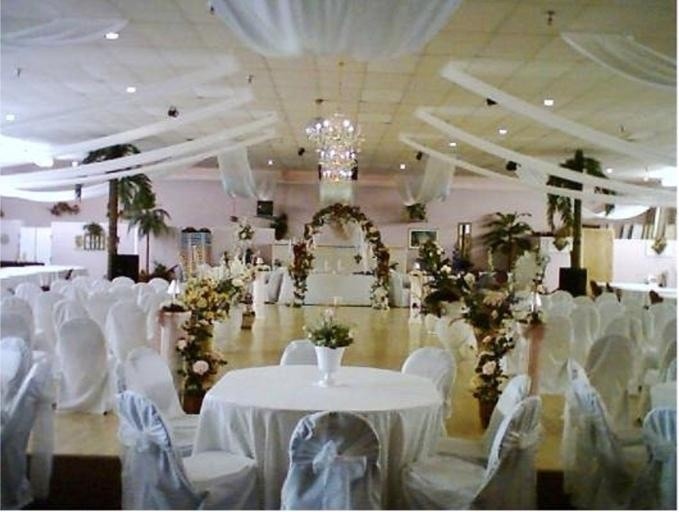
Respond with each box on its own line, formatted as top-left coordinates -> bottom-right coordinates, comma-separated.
314,346 -> 346,388
242,313 -> 254,329
184,394 -> 201,414
478,402 -> 496,426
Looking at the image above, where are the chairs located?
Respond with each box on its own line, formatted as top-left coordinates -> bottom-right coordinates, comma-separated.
532,289 -> 677,510
273,412 -> 384,507
125,345 -> 199,458
403,347 -> 457,418
115,391 -> 261,511
281,340 -> 317,365
199,264 -> 284,303
441,374 -> 532,468
403,395 -> 539,509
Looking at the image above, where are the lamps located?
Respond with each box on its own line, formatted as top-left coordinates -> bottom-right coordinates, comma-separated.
305,112 -> 365,182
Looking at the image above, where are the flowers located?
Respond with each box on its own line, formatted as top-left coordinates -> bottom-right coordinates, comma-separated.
239,294 -> 254,314
302,308 -> 353,350
288,202 -> 397,310
412,211 -> 550,398
176,276 -> 243,390
